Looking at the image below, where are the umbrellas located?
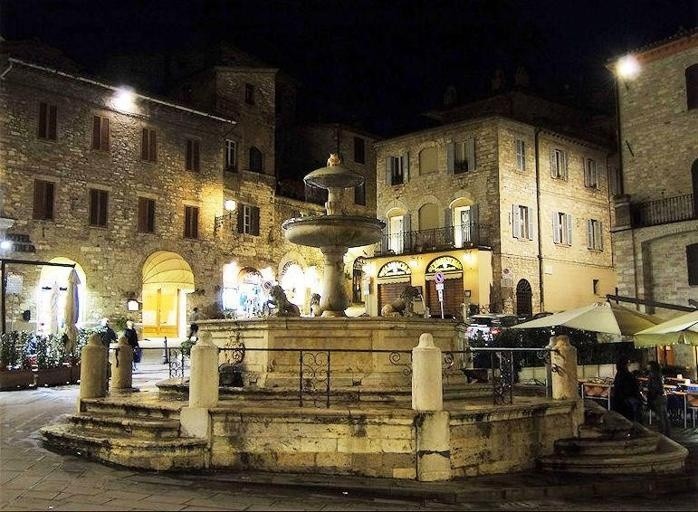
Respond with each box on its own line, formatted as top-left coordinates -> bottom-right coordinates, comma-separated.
633,307 -> 698,347
60,269 -> 81,355
49,284 -> 60,337
507,292 -> 698,338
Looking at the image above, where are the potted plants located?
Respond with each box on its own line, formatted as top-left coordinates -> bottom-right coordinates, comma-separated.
1,329 -> 111,389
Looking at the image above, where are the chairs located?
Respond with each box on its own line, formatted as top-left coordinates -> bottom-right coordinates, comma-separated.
579,376 -> 697,430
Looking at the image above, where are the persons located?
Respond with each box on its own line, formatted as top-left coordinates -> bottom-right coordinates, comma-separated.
188,307 -> 200,341
647,359 -> 672,436
122,320 -> 140,371
613,360 -> 646,425
97,318 -> 117,343
